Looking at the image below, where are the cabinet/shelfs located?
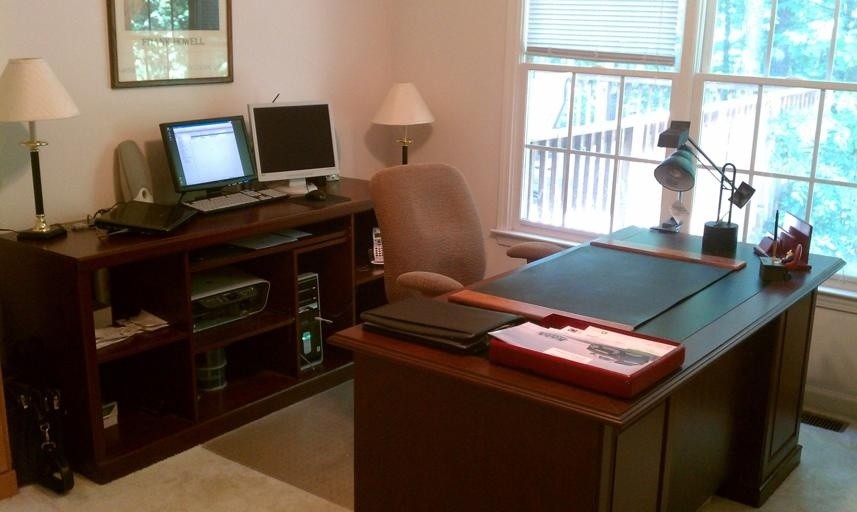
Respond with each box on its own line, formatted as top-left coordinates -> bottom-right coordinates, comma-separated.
0,176 -> 385,483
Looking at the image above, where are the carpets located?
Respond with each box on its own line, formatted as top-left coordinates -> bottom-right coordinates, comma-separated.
201,380 -> 354,511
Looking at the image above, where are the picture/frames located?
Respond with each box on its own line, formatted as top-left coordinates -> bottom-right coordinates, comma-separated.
106,0 -> 233,90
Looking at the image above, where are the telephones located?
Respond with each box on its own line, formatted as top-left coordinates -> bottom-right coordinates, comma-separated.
370,227 -> 385,265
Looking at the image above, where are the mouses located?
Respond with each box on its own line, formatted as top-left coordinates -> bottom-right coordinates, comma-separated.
304,190 -> 327,201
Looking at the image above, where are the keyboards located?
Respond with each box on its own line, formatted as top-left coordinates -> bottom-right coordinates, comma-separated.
181,188 -> 288,216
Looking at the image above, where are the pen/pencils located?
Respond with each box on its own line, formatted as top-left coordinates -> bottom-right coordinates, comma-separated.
772,209 -> 778,259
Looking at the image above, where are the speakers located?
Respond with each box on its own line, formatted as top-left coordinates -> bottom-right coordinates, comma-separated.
117,140 -> 155,204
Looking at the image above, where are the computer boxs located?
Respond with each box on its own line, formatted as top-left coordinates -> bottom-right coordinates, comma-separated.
296,271 -> 324,371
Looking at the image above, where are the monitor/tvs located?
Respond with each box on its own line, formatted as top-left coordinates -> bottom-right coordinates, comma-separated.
159,115 -> 257,199
248,100 -> 339,198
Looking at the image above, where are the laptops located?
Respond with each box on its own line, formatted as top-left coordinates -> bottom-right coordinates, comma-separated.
96,202 -> 199,232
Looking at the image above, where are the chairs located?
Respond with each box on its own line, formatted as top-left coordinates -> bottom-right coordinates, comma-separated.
370,162 -> 566,299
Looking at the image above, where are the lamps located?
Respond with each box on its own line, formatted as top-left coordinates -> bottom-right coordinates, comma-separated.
0,56 -> 81,245
371,81 -> 434,165
654,146 -> 697,234
657,116 -> 747,256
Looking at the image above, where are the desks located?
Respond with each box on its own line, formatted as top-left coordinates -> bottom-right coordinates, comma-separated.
326,224 -> 846,511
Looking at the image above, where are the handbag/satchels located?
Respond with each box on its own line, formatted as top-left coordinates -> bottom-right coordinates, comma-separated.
9,381 -> 67,485
